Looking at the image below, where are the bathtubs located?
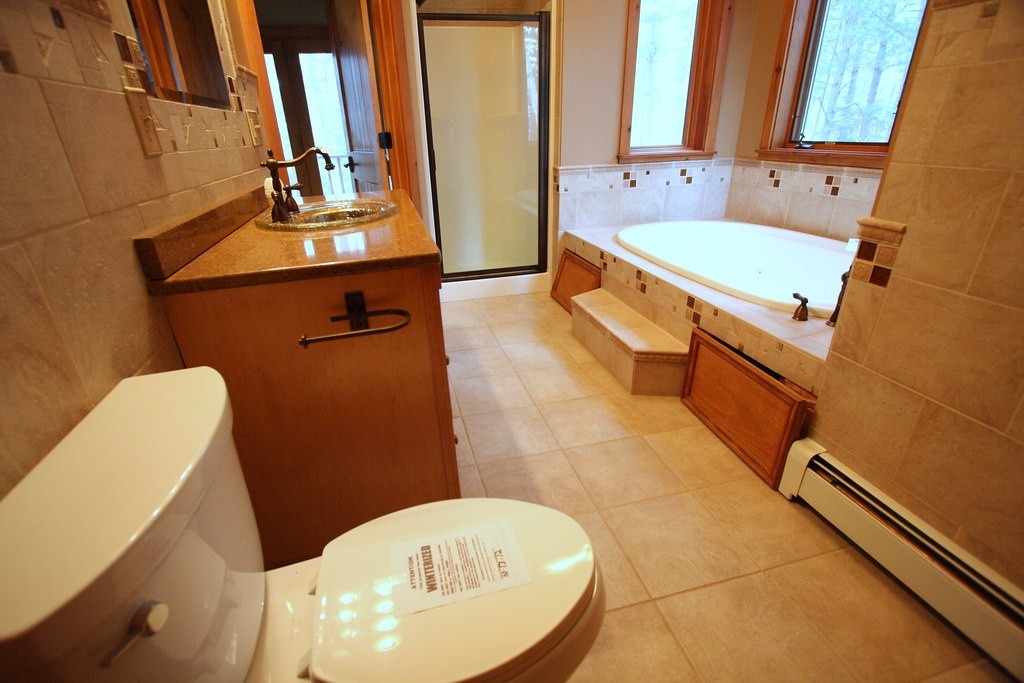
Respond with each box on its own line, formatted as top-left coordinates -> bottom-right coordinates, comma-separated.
561,218 -> 858,437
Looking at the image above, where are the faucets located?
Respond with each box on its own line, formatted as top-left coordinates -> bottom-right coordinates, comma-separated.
260,146 -> 335,212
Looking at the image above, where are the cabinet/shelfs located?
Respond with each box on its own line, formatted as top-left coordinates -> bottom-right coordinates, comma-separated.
676,326 -> 818,489
160,263 -> 463,573
549,247 -> 602,317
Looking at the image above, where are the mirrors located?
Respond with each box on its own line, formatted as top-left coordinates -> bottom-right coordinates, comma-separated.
127,0 -> 232,112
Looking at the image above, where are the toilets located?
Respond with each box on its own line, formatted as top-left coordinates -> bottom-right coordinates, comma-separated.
0,363 -> 606,683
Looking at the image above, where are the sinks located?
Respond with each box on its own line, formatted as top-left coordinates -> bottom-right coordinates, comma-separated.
292,205 -> 378,223
